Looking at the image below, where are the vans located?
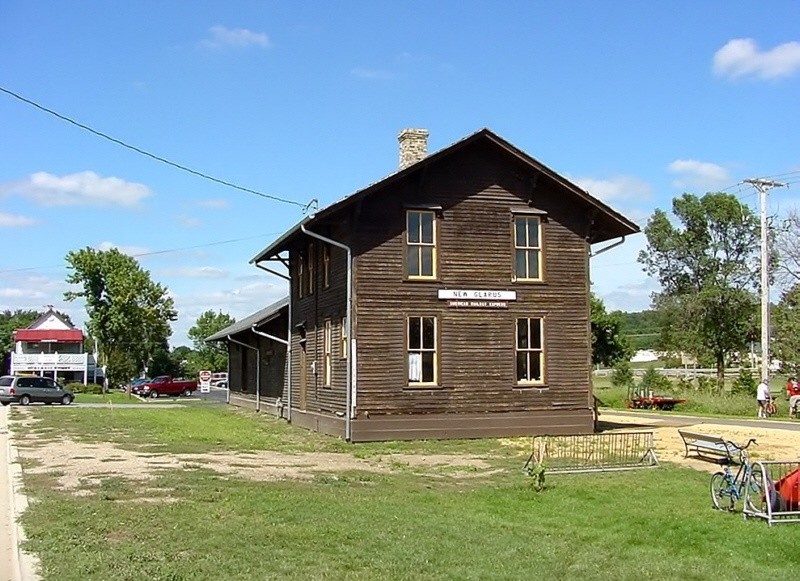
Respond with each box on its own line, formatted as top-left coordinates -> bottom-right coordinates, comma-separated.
0,375 -> 75,406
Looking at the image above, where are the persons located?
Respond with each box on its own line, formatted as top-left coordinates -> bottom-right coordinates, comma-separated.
756,379 -> 774,418
785,374 -> 800,421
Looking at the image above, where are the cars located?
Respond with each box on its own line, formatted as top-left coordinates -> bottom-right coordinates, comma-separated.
217,382 -> 227,388
121,379 -> 151,395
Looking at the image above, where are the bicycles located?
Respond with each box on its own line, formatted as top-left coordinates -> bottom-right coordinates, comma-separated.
709,437 -> 776,513
766,397 -> 776,414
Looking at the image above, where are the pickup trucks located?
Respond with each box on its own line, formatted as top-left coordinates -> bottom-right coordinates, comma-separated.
144,376 -> 198,399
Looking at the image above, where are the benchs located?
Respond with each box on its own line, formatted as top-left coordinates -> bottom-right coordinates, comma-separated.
679,429 -> 757,466
